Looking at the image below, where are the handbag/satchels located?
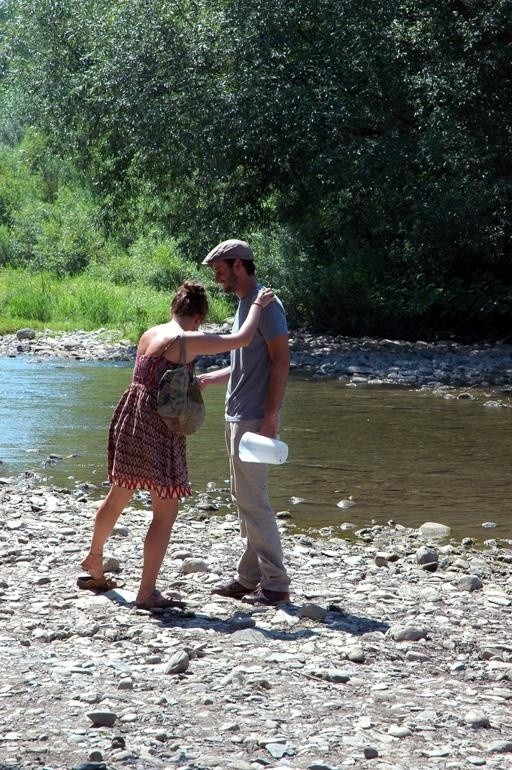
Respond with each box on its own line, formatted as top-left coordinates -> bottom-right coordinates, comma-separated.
156,331 -> 206,436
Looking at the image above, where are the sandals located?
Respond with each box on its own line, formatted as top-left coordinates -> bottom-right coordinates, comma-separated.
77,573 -> 117,590
135,590 -> 187,609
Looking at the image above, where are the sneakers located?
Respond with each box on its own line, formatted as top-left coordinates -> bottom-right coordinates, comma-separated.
212,579 -> 255,597
242,585 -> 290,605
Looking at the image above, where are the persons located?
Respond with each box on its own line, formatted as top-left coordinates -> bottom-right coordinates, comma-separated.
191,239 -> 293,604
79,282 -> 276,613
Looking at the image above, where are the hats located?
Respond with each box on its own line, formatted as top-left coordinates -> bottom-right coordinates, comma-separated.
201,238 -> 254,265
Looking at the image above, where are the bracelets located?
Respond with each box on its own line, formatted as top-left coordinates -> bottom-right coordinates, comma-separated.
251,301 -> 263,309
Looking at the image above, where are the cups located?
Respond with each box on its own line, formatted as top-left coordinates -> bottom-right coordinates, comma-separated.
236,428 -> 288,467
